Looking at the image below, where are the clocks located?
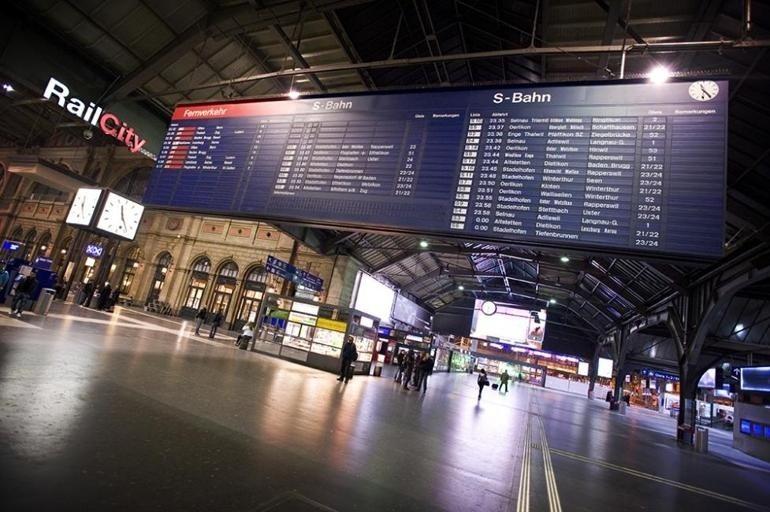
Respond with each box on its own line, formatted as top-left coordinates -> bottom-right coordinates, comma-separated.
686,80 -> 721,102
53,186 -> 146,309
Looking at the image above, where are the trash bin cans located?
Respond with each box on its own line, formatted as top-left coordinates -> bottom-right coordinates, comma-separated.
589,391 -> 595,400
696,427 -> 708,453
619,402 -> 627,415
374,365 -> 382,376
34,288 -> 56,316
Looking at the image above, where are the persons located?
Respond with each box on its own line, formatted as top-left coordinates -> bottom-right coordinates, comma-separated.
478,369 -> 487,398
394,349 -> 434,393
235,321 -> 253,346
499,370 -> 509,392
0,262 -> 9,298
337,335 -> 356,384
8,270 -> 38,317
610,396 -> 627,409
209,309 -> 223,338
80,279 -> 120,313
193,305 -> 207,336
